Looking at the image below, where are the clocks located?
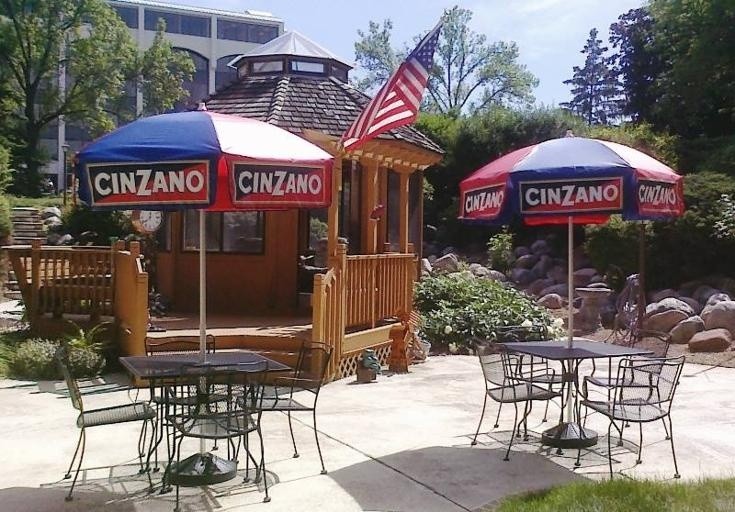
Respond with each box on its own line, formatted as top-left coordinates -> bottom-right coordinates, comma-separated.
131,209 -> 163,234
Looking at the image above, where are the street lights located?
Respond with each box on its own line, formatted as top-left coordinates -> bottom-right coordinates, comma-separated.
60,141 -> 70,205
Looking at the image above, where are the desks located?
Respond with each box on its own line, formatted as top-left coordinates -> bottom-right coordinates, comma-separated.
118,349 -> 295,494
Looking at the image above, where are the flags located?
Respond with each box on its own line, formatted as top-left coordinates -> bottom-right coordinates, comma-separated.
341,19 -> 442,153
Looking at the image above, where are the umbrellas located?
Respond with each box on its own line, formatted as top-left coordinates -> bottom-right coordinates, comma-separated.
459,130 -> 686,449
74,101 -> 334,486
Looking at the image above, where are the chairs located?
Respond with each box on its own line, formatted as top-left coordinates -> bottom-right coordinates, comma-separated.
233,338 -> 336,483
143,332 -> 241,472
166,361 -> 271,511
299,236 -> 349,293
57,335 -> 158,503
471,322 -> 687,480
